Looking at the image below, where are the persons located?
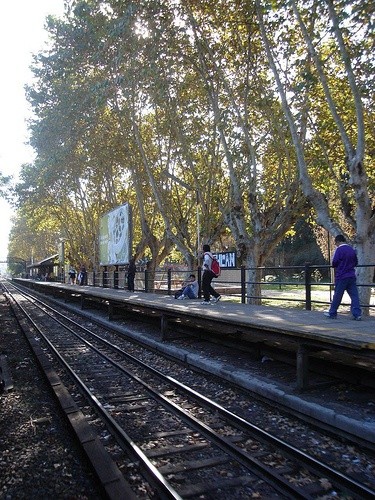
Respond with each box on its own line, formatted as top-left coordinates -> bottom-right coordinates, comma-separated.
77,270 -> 85,286
324,235 -> 362,321
68,268 -> 76,285
200,244 -> 221,305
48,277 -> 52,282
34,274 -> 42,281
177,274 -> 198,300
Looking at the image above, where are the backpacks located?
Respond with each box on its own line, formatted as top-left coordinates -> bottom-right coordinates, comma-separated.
203,253 -> 220,278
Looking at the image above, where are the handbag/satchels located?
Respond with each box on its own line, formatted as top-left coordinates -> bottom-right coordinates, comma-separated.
174,290 -> 183,299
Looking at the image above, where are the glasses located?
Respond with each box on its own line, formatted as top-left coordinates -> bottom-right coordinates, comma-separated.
333,242 -> 337,245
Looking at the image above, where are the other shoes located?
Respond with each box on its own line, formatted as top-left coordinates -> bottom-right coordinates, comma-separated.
201,300 -> 211,305
349,315 -> 362,320
177,296 -> 185,300
323,312 -> 337,319
214,296 -> 221,303
